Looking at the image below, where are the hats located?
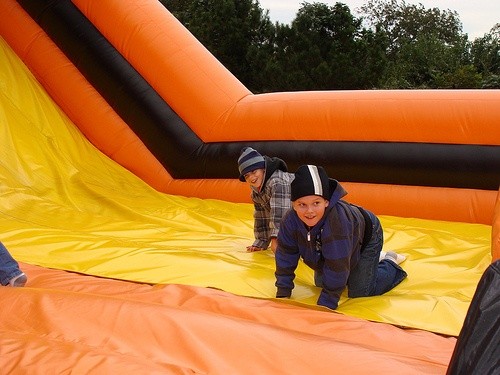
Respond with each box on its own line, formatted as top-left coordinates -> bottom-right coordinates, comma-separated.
238,147 -> 265,182
291,165 -> 330,201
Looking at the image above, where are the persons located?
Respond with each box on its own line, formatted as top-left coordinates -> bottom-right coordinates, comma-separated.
237,147 -> 297,254
0,241 -> 28,286
273,164 -> 409,309
